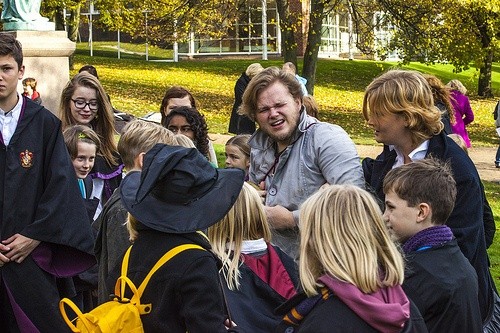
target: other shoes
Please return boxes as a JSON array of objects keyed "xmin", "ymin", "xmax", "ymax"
[{"xmin": 494, "ymin": 161, "xmax": 500, "ymax": 168}]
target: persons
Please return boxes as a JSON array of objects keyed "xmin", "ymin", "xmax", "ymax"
[
  {"xmin": 241, "ymin": 66, "xmax": 366, "ymax": 263},
  {"xmin": 164, "ymin": 106, "xmax": 210, "ymax": 161},
  {"xmin": 382, "ymin": 159, "xmax": 484, "ymax": 333},
  {"xmin": 160, "ymin": 87, "xmax": 218, "ymax": 168},
  {"xmin": 207, "ymin": 181, "xmax": 304, "ymax": 333},
  {"xmin": 362, "ymin": 70, "xmax": 495, "ymax": 325},
  {"xmin": 92, "ymin": 120, "xmax": 195, "ymax": 305},
  {"xmin": 60, "ymin": 65, "xmax": 126, "ymax": 225},
  {"xmin": 119, "ymin": 143, "xmax": 245, "ymax": 333},
  {"xmin": 494, "ymin": 100, "xmax": 500, "ymax": 167},
  {"xmin": 226, "ymin": 135, "xmax": 253, "ymax": 181},
  {"xmin": 228, "ymin": 63, "xmax": 264, "ymax": 134},
  {"xmin": 64, "ymin": 125, "xmax": 100, "ymax": 199},
  {"xmin": 274, "ymin": 184, "xmax": 429, "ymax": 333},
  {"xmin": 283, "ymin": 61, "xmax": 308, "ymax": 96},
  {"xmin": 22, "ymin": 78, "xmax": 42, "ymax": 106},
  {"xmin": 447, "ymin": 79, "xmax": 474, "ymax": 147},
  {"xmin": 0, "ymin": 33, "xmax": 99, "ymax": 333}
]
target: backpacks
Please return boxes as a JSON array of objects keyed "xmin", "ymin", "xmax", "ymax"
[
  {"xmin": 59, "ymin": 243, "xmax": 208, "ymax": 333},
  {"xmin": 492, "ymin": 101, "xmax": 499, "ymax": 120}
]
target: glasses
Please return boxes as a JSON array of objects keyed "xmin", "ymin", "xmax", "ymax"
[{"xmin": 71, "ymin": 97, "xmax": 100, "ymax": 110}]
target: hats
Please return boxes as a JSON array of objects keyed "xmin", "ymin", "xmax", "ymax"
[{"xmin": 119, "ymin": 143, "xmax": 246, "ymax": 234}]
